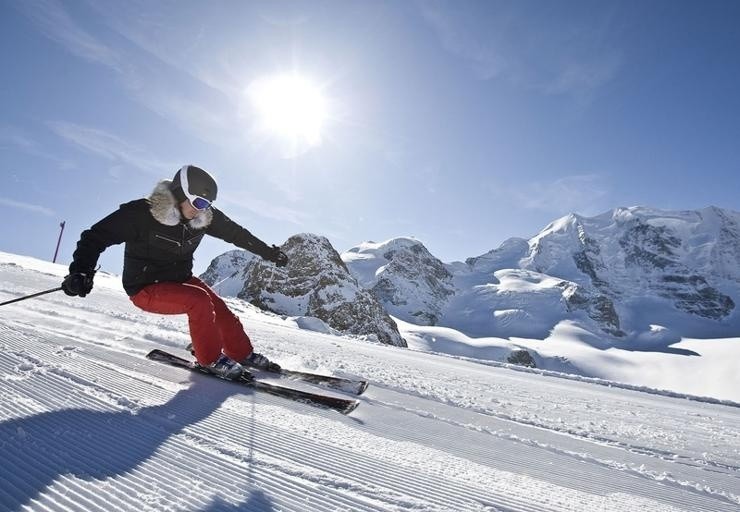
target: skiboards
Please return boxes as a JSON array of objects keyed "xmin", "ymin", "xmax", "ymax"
[{"xmin": 146, "ymin": 343, "xmax": 369, "ymax": 415}]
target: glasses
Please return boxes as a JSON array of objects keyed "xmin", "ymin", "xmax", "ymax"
[{"xmin": 188, "ymin": 194, "xmax": 213, "ymax": 211}]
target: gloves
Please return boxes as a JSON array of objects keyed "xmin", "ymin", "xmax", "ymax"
[
  {"xmin": 63, "ymin": 264, "xmax": 97, "ymax": 298},
  {"xmin": 270, "ymin": 244, "xmax": 289, "ymax": 268}
]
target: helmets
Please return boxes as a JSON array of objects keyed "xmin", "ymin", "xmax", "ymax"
[{"xmin": 170, "ymin": 166, "xmax": 217, "ymax": 204}]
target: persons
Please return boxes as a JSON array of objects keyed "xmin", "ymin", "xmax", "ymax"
[{"xmin": 61, "ymin": 162, "xmax": 290, "ymax": 382}]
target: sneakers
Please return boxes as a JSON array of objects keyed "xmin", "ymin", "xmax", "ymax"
[
  {"xmin": 239, "ymin": 352, "xmax": 270, "ymax": 371},
  {"xmin": 198, "ymin": 352, "xmax": 244, "ymax": 381}
]
